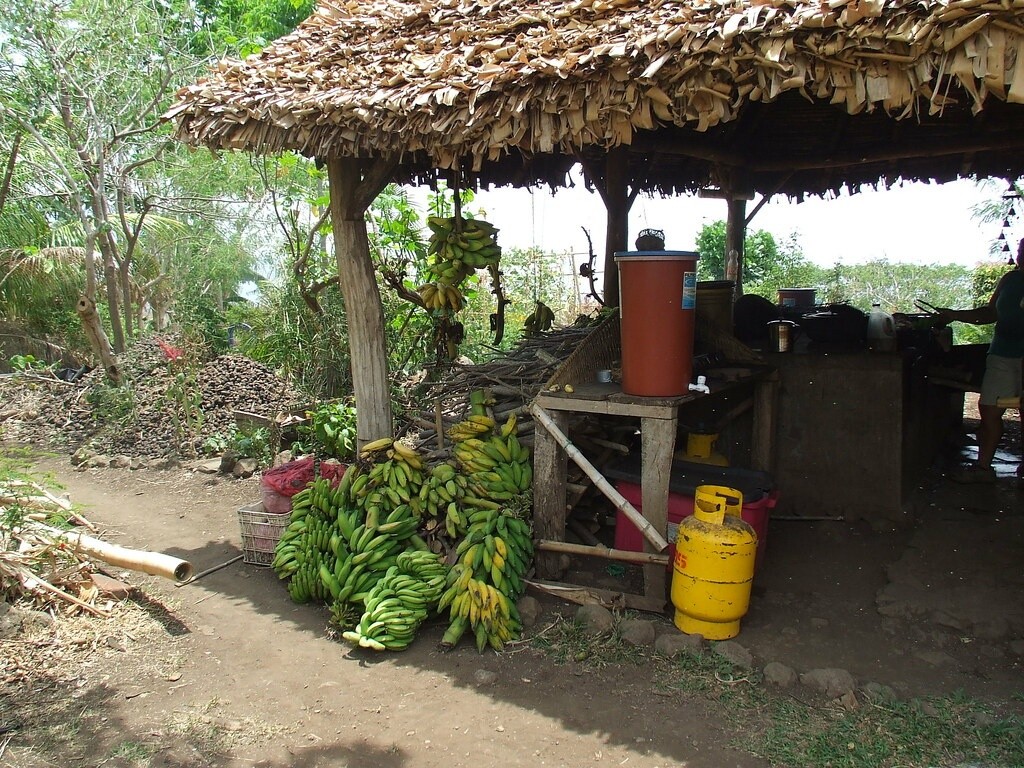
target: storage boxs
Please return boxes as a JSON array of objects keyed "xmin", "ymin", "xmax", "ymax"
[
  {"xmin": 601, "ymin": 450, "xmax": 783, "ymax": 572},
  {"xmin": 236, "ymin": 501, "xmax": 293, "ymax": 566}
]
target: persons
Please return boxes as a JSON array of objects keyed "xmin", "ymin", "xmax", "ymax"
[{"xmin": 935, "ymin": 237, "xmax": 1024, "ymax": 484}]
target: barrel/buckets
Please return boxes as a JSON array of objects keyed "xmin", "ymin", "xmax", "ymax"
[
  {"xmin": 613, "ymin": 251, "xmax": 700, "ymax": 396},
  {"xmin": 696, "ymin": 288, "xmax": 735, "ymax": 334}
]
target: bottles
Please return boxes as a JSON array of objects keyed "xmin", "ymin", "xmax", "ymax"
[{"xmin": 867, "ymin": 303, "xmax": 896, "ymax": 353}]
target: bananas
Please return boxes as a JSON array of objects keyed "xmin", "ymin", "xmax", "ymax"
[
  {"xmin": 271, "ymin": 414, "xmax": 537, "ymax": 653},
  {"xmin": 416, "ymin": 216, "xmax": 500, "ymax": 314},
  {"xmin": 549, "ymin": 383, "xmax": 574, "ymax": 393}
]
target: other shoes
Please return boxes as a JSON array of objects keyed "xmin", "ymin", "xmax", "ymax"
[{"xmin": 951, "ymin": 464, "xmax": 996, "ymax": 483}]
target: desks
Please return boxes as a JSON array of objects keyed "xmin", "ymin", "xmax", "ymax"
[{"xmin": 530, "ymin": 372, "xmax": 778, "ymax": 616}]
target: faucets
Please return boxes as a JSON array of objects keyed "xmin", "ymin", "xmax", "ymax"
[{"xmin": 689, "ymin": 373, "xmax": 710, "ymax": 395}]
